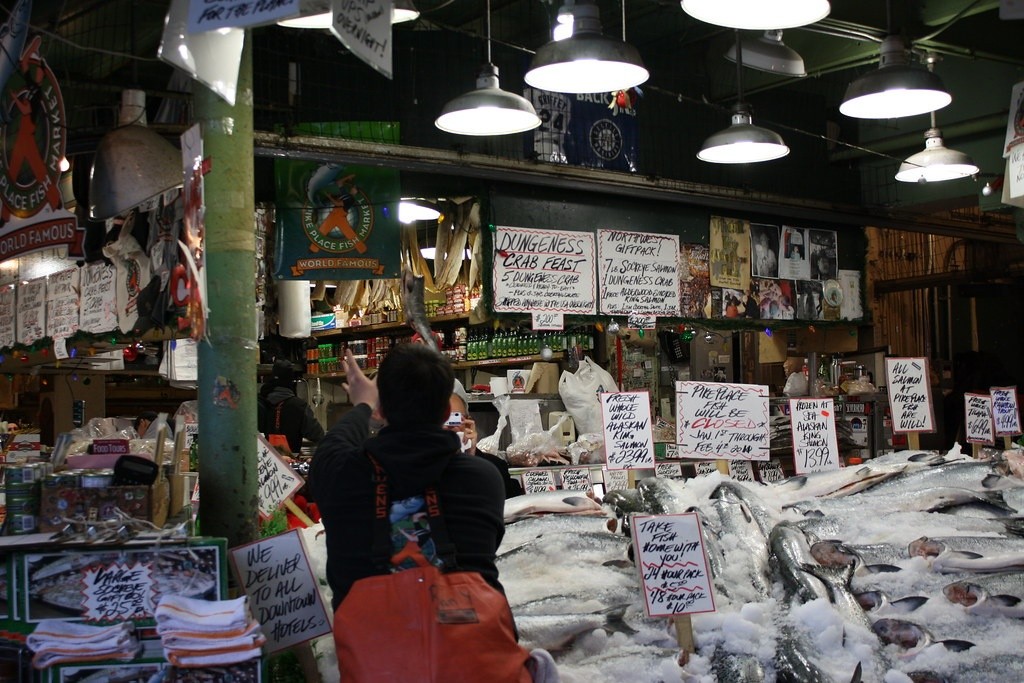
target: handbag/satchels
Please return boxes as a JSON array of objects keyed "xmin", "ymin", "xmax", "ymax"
[{"xmin": 558, "ymin": 355, "xmax": 619, "ymax": 437}]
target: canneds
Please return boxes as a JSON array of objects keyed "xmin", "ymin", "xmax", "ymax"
[
  {"xmin": 4, "ymin": 462, "xmax": 113, "ymax": 535},
  {"xmin": 305, "ymin": 346, "xmax": 320, "ymax": 376},
  {"xmin": 366, "ymin": 336, "xmax": 389, "ymax": 368},
  {"xmin": 435, "ymin": 326, "xmax": 467, "ymax": 362}
]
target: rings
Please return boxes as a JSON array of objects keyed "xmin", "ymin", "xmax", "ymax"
[{"xmin": 469, "ymin": 429, "xmax": 473, "ymax": 434}]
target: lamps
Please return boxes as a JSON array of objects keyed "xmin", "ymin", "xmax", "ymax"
[
  {"xmin": 275, "ymin": 0, "xmax": 421, "ymax": 29},
  {"xmin": 838, "ymin": 0, "xmax": 952, "ymax": 119},
  {"xmin": 721, "ymin": 27, "xmax": 807, "ymax": 77},
  {"xmin": 433, "ymin": 0, "xmax": 542, "ymax": 137},
  {"xmin": 522, "ymin": 0, "xmax": 650, "ymax": 94},
  {"xmin": 695, "ymin": 29, "xmax": 791, "ymax": 164},
  {"xmin": 680, "ymin": 0, "xmax": 831, "ymax": 30},
  {"xmin": 894, "ymin": 52, "xmax": 980, "ymax": 184}
]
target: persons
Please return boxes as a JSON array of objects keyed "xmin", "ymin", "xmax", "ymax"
[
  {"xmin": 308, "ymin": 343, "xmax": 521, "ymax": 639},
  {"xmin": 7, "ymin": 422, "xmax": 20, "ymax": 432},
  {"xmin": 443, "ymin": 379, "xmax": 525, "ymax": 497},
  {"xmin": 322, "ymin": 172, "xmax": 360, "ymax": 213},
  {"xmin": 679, "ymin": 233, "xmax": 831, "ymax": 317},
  {"xmin": 134, "ymin": 411, "xmax": 173, "ymax": 439},
  {"xmin": 258, "ymin": 358, "xmax": 325, "ymax": 452}
]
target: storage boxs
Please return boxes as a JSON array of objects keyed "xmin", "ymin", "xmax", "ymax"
[
  {"xmin": 88, "ymin": 349, "xmax": 124, "ymax": 370},
  {"xmin": 4, "ymin": 433, "xmax": 41, "ymax": 464},
  {"xmin": 506, "ymin": 369, "xmax": 531, "ymax": 393},
  {"xmin": 311, "ymin": 283, "xmax": 481, "ymax": 332}
]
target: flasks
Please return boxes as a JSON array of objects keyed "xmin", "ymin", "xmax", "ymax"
[{"xmin": 852, "ymin": 361, "xmax": 868, "ymax": 381}]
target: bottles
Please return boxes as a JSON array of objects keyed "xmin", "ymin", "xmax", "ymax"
[
  {"xmin": 466, "ymin": 324, "xmax": 594, "ymax": 361},
  {"xmin": 801, "ymin": 358, "xmax": 809, "ymax": 381},
  {"xmin": 818, "ymin": 352, "xmax": 843, "ymax": 389}
]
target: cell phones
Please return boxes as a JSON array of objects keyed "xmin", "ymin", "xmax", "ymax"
[{"xmin": 444, "ymin": 412, "xmax": 462, "ymax": 426}]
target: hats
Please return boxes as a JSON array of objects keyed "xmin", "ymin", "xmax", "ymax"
[{"xmin": 453, "ymin": 377, "xmax": 469, "ymax": 414}]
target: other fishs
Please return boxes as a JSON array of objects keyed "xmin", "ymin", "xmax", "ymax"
[{"xmin": 492, "ymin": 449, "xmax": 1024, "ymax": 683}]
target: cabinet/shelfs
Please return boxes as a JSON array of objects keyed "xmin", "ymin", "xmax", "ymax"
[{"xmin": 302, "ymin": 312, "xmax": 595, "ymax": 379}]
